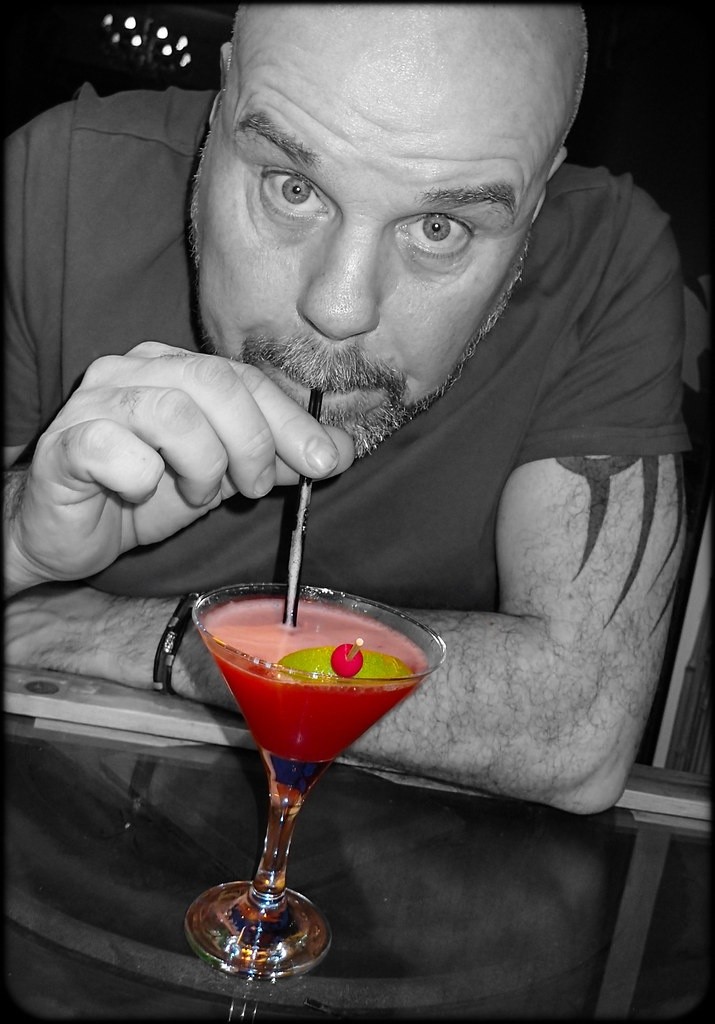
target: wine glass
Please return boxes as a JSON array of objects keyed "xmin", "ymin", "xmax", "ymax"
[{"xmin": 182, "ymin": 584, "xmax": 446, "ymax": 975}]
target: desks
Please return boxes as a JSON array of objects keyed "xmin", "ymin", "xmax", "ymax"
[{"xmin": 0, "ymin": 716, "xmax": 715, "ymax": 1024}]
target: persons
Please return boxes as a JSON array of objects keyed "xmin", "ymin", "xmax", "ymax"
[{"xmin": 0, "ymin": 0, "xmax": 694, "ymax": 815}]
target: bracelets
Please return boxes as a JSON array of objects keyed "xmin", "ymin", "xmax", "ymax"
[{"xmin": 148, "ymin": 586, "xmax": 210, "ymax": 704}]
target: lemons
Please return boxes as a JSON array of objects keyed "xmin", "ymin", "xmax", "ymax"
[{"xmin": 273, "ymin": 645, "xmax": 416, "ymax": 685}]
